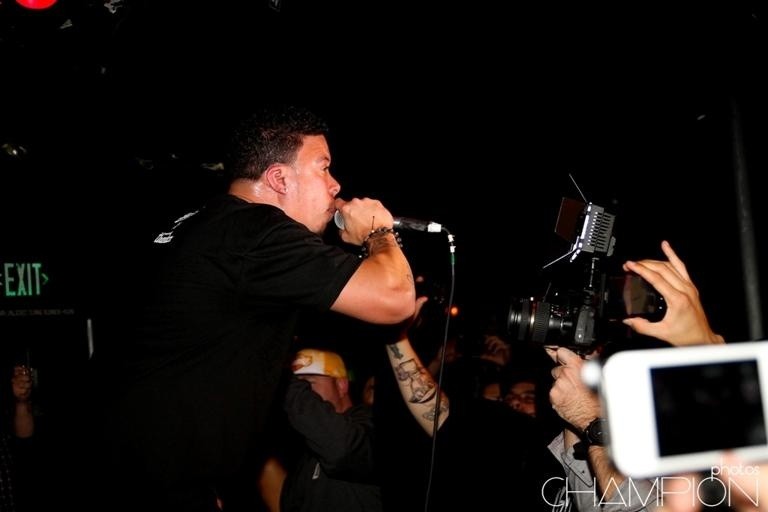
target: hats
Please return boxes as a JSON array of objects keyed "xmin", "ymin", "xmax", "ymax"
[{"xmin": 285, "ymin": 346, "xmax": 349, "ymax": 380}]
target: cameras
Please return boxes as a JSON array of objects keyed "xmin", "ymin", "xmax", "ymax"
[
  {"xmin": 506, "ymin": 199, "xmax": 668, "ymax": 356},
  {"xmin": 596, "ymin": 341, "xmax": 768, "ymax": 480}
]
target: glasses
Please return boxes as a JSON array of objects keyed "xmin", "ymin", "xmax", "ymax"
[{"xmin": 496, "ymin": 390, "xmax": 539, "ymax": 407}]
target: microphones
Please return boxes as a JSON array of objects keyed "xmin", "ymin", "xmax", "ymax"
[{"xmin": 333, "ymin": 208, "xmax": 443, "ymax": 239}]
